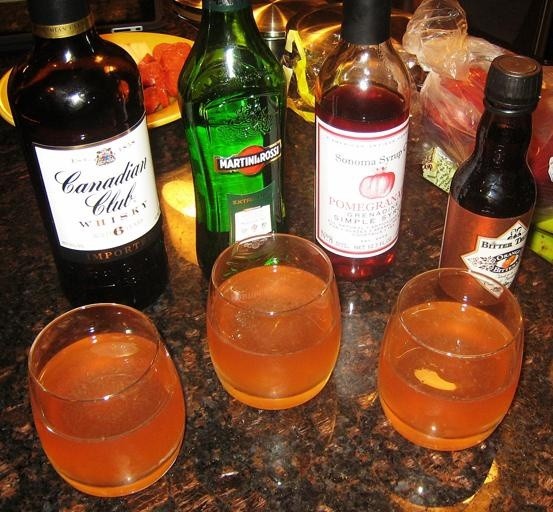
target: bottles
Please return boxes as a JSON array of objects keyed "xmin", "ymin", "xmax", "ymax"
[
  {"xmin": 434, "ymin": 51, "xmax": 551, "ymax": 313},
  {"xmin": 177, "ymin": 1, "xmax": 288, "ymax": 282},
  {"xmin": 8, "ymin": 2, "xmax": 176, "ymax": 312}
]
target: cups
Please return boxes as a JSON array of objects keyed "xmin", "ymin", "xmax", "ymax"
[
  {"xmin": 314, "ymin": 2, "xmax": 408, "ymax": 283},
  {"xmin": 25, "ymin": 300, "xmax": 190, "ymax": 502},
  {"xmin": 201, "ymin": 232, "xmax": 346, "ymax": 415},
  {"xmin": 377, "ymin": 268, "xmax": 525, "ymax": 456}
]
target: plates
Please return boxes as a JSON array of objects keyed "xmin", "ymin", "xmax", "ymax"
[{"xmin": 1, "ymin": 29, "xmax": 195, "ymax": 132}]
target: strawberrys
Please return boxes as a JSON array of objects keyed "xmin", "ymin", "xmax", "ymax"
[{"xmin": 138, "ymin": 43, "xmax": 193, "ymax": 115}]
